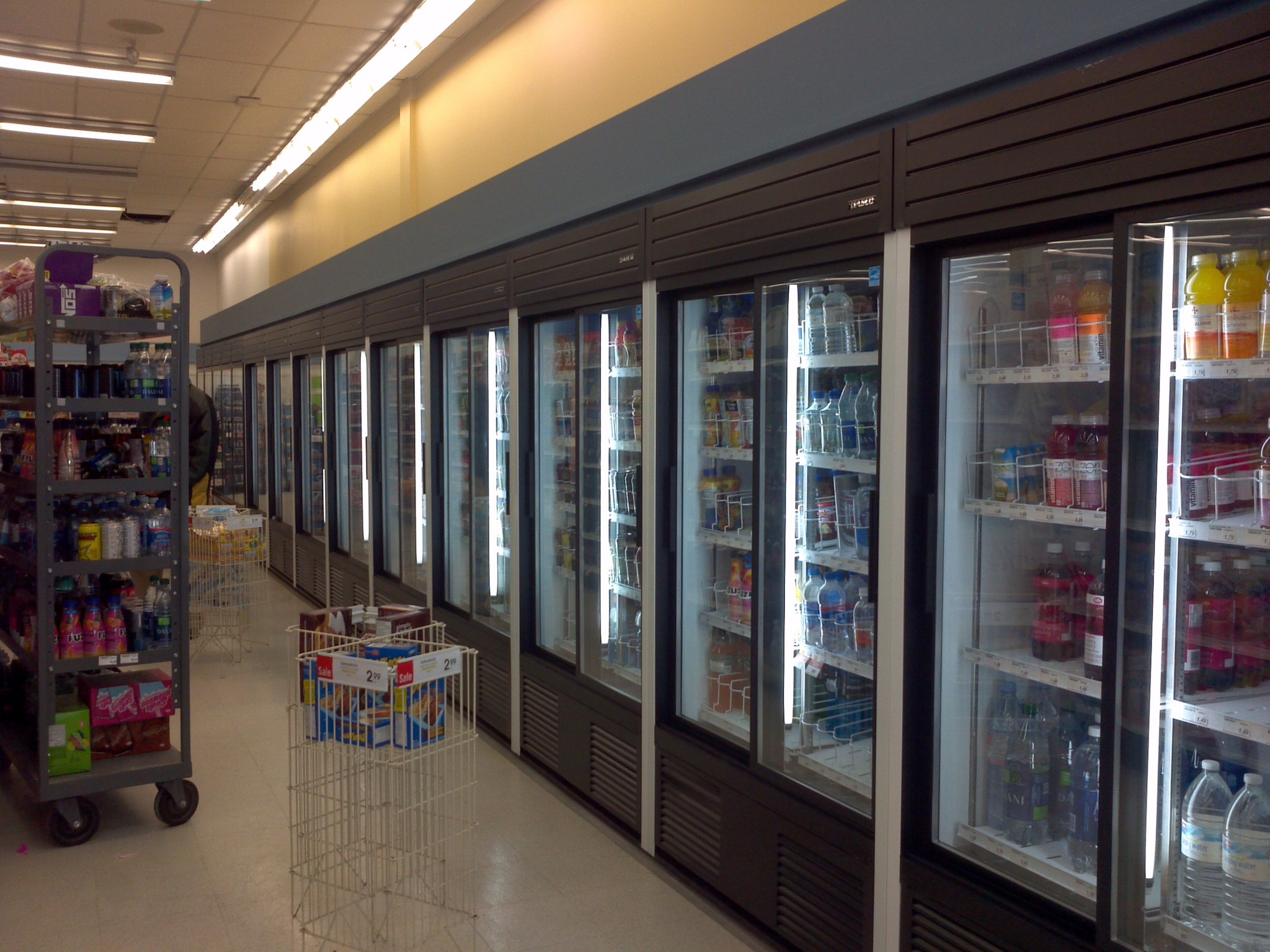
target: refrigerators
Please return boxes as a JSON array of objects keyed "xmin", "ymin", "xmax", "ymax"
[
  {"xmin": 654, "ymin": 234, "xmax": 885, "ymax": 951},
  {"xmin": 211, "ymin": 314, "xmax": 645, "ymax": 838},
  {"xmin": 899, "ymin": 194, "xmax": 1270, "ymax": 949}
]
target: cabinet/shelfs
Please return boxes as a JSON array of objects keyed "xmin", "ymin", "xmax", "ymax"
[{"xmin": 0, "ymin": 244, "xmax": 192, "ymax": 802}]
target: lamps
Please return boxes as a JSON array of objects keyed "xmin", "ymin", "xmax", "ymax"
[{"xmin": 0, "ymin": 43, "xmax": 176, "ymax": 236}]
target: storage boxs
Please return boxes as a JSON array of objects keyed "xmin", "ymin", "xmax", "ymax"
[
  {"xmin": 16, "ymin": 241, "xmax": 101, "ymax": 317},
  {"xmin": 304, "ymin": 643, "xmax": 443, "ymax": 749},
  {"xmin": 50, "ymin": 669, "xmax": 175, "ymax": 776}
]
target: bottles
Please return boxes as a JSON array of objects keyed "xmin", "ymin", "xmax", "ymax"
[
  {"xmin": 0, "ymin": 273, "xmax": 174, "ymax": 664},
  {"xmin": 981, "ymin": 248, "xmax": 1270, "ymax": 952},
  {"xmin": 188, "ymin": 505, "xmax": 194, "ymax": 529},
  {"xmin": 698, "ymin": 283, "xmax": 882, "ymax": 714}
]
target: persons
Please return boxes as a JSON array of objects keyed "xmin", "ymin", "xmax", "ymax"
[{"xmin": 140, "ymin": 377, "xmax": 219, "ymax": 512}]
